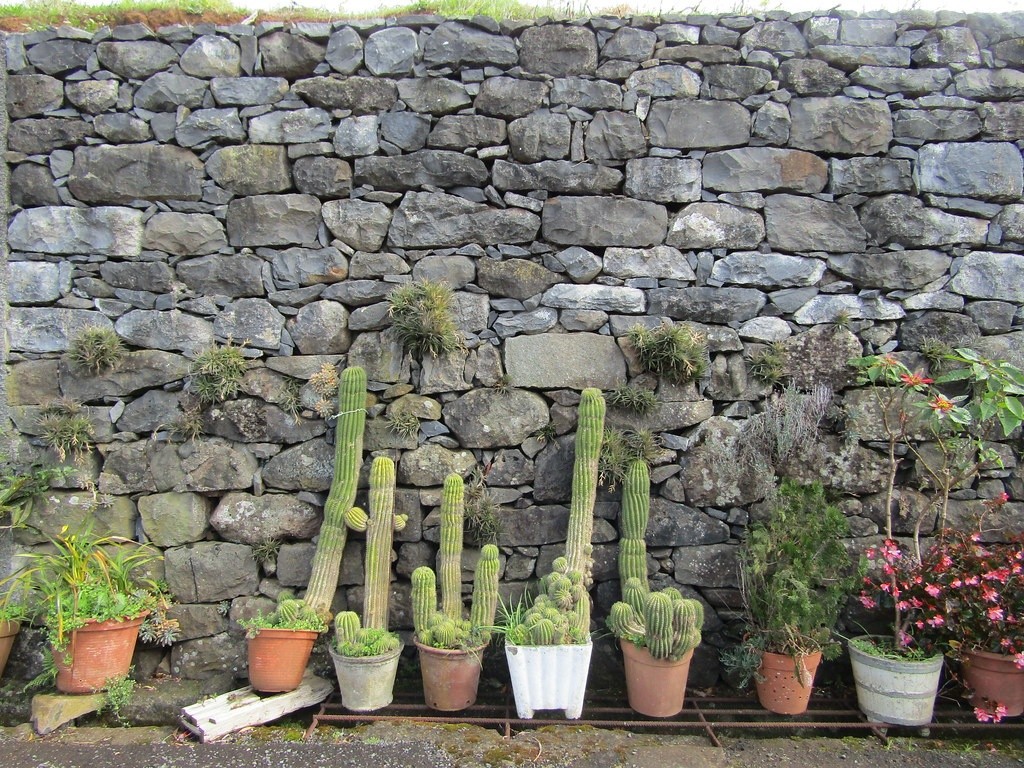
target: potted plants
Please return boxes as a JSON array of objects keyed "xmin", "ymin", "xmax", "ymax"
[
  {"xmin": 329, "ymin": 457, "xmax": 406, "ymax": 713},
  {"xmin": 720, "ymin": 477, "xmax": 867, "ymax": 715},
  {"xmin": 606, "ymin": 460, "xmax": 704, "ymax": 718},
  {"xmin": 0, "ymin": 516, "xmax": 182, "ymax": 696},
  {"xmin": 234, "ymin": 366, "xmax": 366, "ymax": 691},
  {"xmin": 477, "ymin": 388, "xmax": 605, "ymax": 718},
  {"xmin": 411, "ymin": 474, "xmax": 500, "ymax": 712},
  {"xmin": 0, "ymin": 603, "xmax": 34, "ymax": 678},
  {"xmin": 832, "ymin": 348, "xmax": 1024, "ymax": 727}
]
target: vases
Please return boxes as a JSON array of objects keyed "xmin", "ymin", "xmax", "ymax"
[{"xmin": 960, "ymin": 644, "xmax": 1024, "ymax": 717}]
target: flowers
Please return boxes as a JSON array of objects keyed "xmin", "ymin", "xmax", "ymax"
[{"xmin": 860, "ymin": 490, "xmax": 1024, "ymax": 723}]
